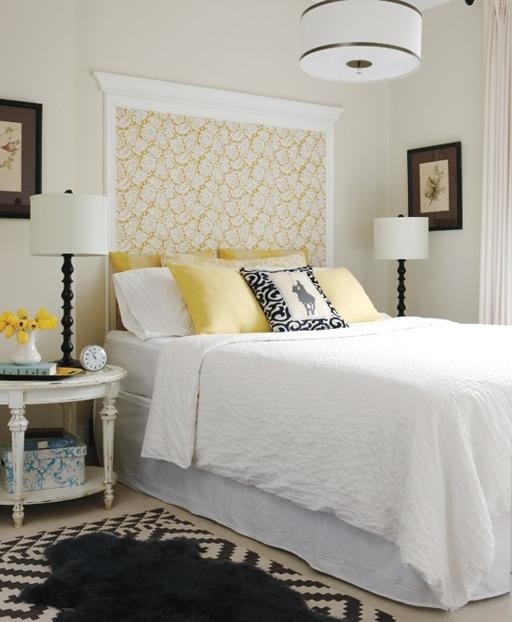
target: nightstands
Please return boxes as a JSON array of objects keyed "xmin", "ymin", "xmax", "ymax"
[{"xmin": 0, "ymin": 364, "xmax": 129, "ymax": 528}]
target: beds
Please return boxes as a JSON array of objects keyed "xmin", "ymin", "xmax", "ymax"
[{"xmin": 97, "ymin": 247, "xmax": 511, "ymax": 610}]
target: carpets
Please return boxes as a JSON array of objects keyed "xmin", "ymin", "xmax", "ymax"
[{"xmin": 0, "ymin": 509, "xmax": 398, "ymax": 622}]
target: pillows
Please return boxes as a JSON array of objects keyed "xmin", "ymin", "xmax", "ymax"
[
  {"xmin": 242, "ymin": 266, "xmax": 347, "ymax": 331},
  {"xmin": 161, "ymin": 254, "xmax": 307, "ymax": 267},
  {"xmin": 167, "ymin": 265, "xmax": 383, "ymax": 333}
]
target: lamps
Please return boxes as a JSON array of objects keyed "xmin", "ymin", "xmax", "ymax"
[
  {"xmin": 372, "ymin": 214, "xmax": 428, "ymax": 317},
  {"xmin": 30, "ymin": 190, "xmax": 108, "ymax": 373},
  {"xmin": 298, "ymin": 1, "xmax": 421, "ymax": 84}
]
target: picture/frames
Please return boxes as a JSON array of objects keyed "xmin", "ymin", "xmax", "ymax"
[
  {"xmin": 2, "ymin": 99, "xmax": 41, "ymax": 219},
  {"xmin": 406, "ymin": 141, "xmax": 462, "ymax": 230}
]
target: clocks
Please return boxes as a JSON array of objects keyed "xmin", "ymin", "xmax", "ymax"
[{"xmin": 79, "ymin": 345, "xmax": 107, "ymax": 371}]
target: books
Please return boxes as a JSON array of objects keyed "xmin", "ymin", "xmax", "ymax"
[
  {"xmin": 0, "ymin": 363, "xmax": 57, "ymax": 375},
  {"xmin": 26, "ymin": 427, "xmax": 65, "ymax": 438},
  {"xmin": 24, "ymin": 430, "xmax": 80, "ymax": 451}
]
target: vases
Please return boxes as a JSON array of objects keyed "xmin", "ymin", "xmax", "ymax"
[{"xmin": 14, "ymin": 331, "xmax": 41, "ymax": 364}]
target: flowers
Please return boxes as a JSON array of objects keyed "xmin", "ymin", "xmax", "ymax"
[{"xmin": 0, "ymin": 308, "xmax": 57, "ymax": 341}]
